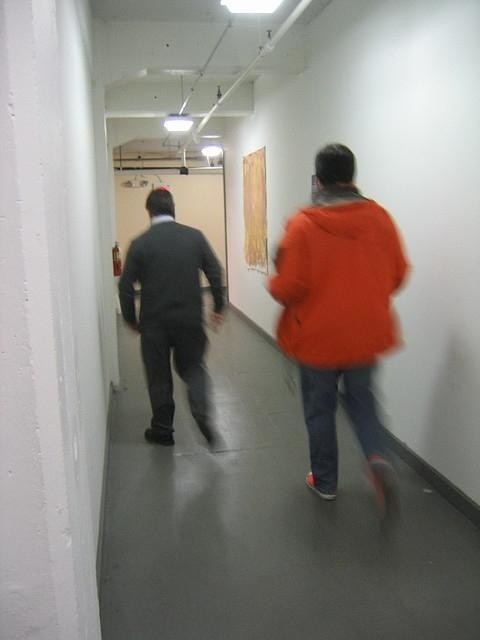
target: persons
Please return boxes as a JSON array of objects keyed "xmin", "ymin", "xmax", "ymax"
[
  {"xmin": 264, "ymin": 144, "xmax": 408, "ymax": 513},
  {"xmin": 118, "ymin": 187, "xmax": 226, "ymax": 449}
]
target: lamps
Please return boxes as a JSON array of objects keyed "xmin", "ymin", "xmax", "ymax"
[
  {"xmin": 200, "ymin": 143, "xmax": 222, "ymax": 155},
  {"xmin": 161, "ymin": 112, "xmax": 194, "ymax": 132}
]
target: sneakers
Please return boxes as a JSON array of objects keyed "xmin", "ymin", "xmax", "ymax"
[
  {"xmin": 370, "ymin": 454, "xmax": 398, "ymax": 495},
  {"xmin": 145, "ymin": 428, "xmax": 174, "ymax": 445},
  {"xmin": 306, "ymin": 472, "xmax": 337, "ymax": 500}
]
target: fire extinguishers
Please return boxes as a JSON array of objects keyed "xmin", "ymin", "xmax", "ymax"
[{"xmin": 112, "ymin": 241, "xmax": 122, "ymax": 276}]
